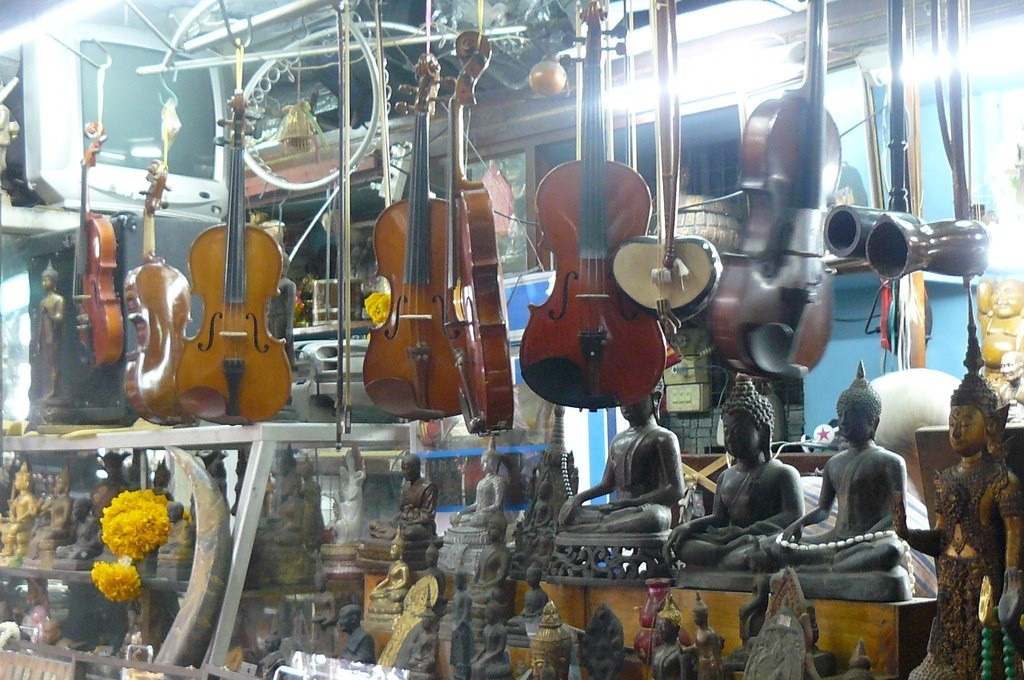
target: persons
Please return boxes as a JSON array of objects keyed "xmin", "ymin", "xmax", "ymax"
[
  {"xmin": 468, "ymin": 601, "xmax": 514, "ymax": 680},
  {"xmin": 457, "ymin": 513, "xmax": 512, "ymax": 643},
  {"xmin": 433, "ymin": 434, "xmax": 505, "ymax": 580},
  {"xmin": 395, "ymin": 586, "xmax": 440, "ymax": 680},
  {"xmin": 544, "ymin": 375, "xmax": 682, "ymax": 575},
  {"xmin": 907, "ymin": 291, "xmax": 1021, "ymax": 679},
  {"xmin": 353, "ymin": 450, "xmax": 438, "ymax": 573},
  {"xmin": 2, "ymin": 442, "xmax": 337, "ymax": 680},
  {"xmin": 33, "ymin": 256, "xmax": 68, "ymax": 404},
  {"xmin": 841, "ymin": 638, "xmax": 875, "ymax": 679},
  {"xmin": 437, "ymin": 572, "xmax": 470, "ymax": 642},
  {"xmin": 339, "ymin": 604, "xmax": 375, "ymax": 665},
  {"xmin": 650, "ymin": 589, "xmax": 683, "ymax": 680},
  {"xmin": 662, "ymin": 372, "xmax": 800, "ymax": 589},
  {"xmin": 775, "ymin": 359, "xmax": 911, "ymax": 607},
  {"xmin": 693, "ymin": 590, "xmax": 723, "ymax": 680},
  {"xmin": 995, "ymin": 352, "xmax": 1023, "ymax": 426},
  {"xmin": 364, "ymin": 525, "xmax": 411, "ymax": 630},
  {"xmin": 973, "ymin": 278, "xmax": 1024, "ymax": 392},
  {"xmin": 502, "ymin": 562, "xmax": 548, "ymax": 648},
  {"xmin": 527, "ymin": 599, "xmax": 570, "ymax": 680}
]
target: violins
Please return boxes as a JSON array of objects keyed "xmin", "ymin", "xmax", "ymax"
[{"xmin": 71, "ymin": 1, "xmax": 844, "ymax": 438}]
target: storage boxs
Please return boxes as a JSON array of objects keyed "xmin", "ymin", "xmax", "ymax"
[{"xmin": 48, "ymin": 0, "xmax": 988, "ymax": 435}]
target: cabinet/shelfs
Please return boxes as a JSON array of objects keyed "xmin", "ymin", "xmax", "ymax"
[{"xmin": 1, "ymin": 423, "xmax": 937, "ymax": 680}]
